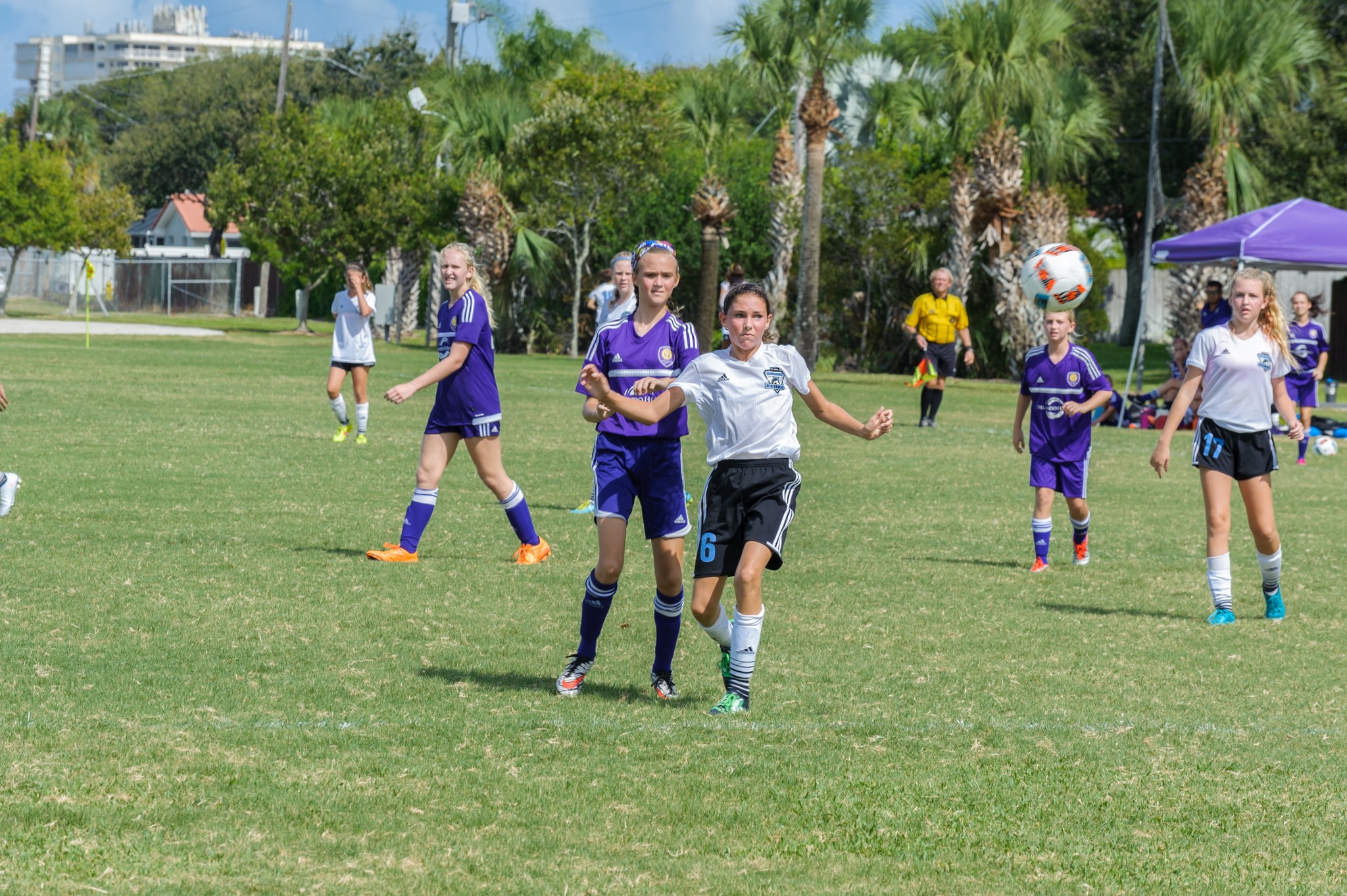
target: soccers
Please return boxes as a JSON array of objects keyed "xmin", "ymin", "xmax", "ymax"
[
  {"xmin": 1017, "ymin": 241, "xmax": 1093, "ymax": 311},
  {"xmin": 1314, "ymin": 435, "xmax": 1338, "ymax": 458}
]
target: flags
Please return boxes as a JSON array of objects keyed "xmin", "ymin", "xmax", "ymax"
[{"xmin": 904, "ymin": 357, "xmax": 938, "ymax": 387}]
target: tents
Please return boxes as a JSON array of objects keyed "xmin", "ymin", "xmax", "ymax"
[{"xmin": 1118, "ymin": 197, "xmax": 1347, "ymax": 427}]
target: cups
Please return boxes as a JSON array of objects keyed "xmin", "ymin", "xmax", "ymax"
[{"xmin": 1326, "ymin": 378, "xmax": 1337, "ymax": 403}]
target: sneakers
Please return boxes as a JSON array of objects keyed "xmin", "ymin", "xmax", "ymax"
[
  {"xmin": 367, "ymin": 542, "xmax": 419, "ymax": 564},
  {"xmin": 1072, "ymin": 534, "xmax": 1090, "ymax": 567},
  {"xmin": 650, "ymin": 670, "xmax": 678, "ymax": 700},
  {"xmin": 512, "ymin": 536, "xmax": 551, "ymax": 565},
  {"xmin": 0, "ymin": 472, "xmax": 21, "ymax": 518},
  {"xmin": 708, "ymin": 687, "xmax": 749, "ymax": 717},
  {"xmin": 1029, "ymin": 556, "xmax": 1050, "ymax": 573},
  {"xmin": 355, "ymin": 433, "xmax": 366, "ymax": 445},
  {"xmin": 332, "ymin": 420, "xmax": 354, "ymax": 443},
  {"xmin": 1206, "ymin": 608, "xmax": 1236, "ymax": 627},
  {"xmin": 1261, "ymin": 582, "xmax": 1285, "ymax": 620},
  {"xmin": 684, "ymin": 491, "xmax": 693, "ymax": 507},
  {"xmin": 569, "ymin": 499, "xmax": 595, "ymax": 514},
  {"xmin": 555, "ymin": 653, "xmax": 595, "ymax": 698},
  {"xmin": 719, "ymin": 619, "xmax": 737, "ymax": 691}
]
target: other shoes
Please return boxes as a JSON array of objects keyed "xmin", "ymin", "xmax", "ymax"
[{"xmin": 1297, "ymin": 458, "xmax": 1306, "ymax": 465}]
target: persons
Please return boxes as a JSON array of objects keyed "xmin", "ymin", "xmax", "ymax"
[
  {"xmin": 557, "ymin": 240, "xmax": 699, "ymax": 701},
  {"xmin": 327, "ymin": 263, "xmax": 376, "ymax": 444},
  {"xmin": 366, "ymin": 243, "xmax": 552, "ymax": 566},
  {"xmin": 1093, "ymin": 280, "xmax": 1234, "ymax": 426},
  {"xmin": 719, "ymin": 263, "xmax": 746, "ymax": 349},
  {"xmin": 570, "ymin": 252, "xmax": 694, "ymax": 513},
  {"xmin": 905, "ymin": 267, "xmax": 974, "ymax": 429},
  {"xmin": 1013, "ymin": 308, "xmax": 1112, "ymax": 572},
  {"xmin": 1149, "ymin": 268, "xmax": 1305, "ymax": 626},
  {"xmin": 0, "ymin": 382, "xmax": 21, "ymax": 515},
  {"xmin": 580, "ymin": 283, "xmax": 893, "ymax": 713},
  {"xmin": 1284, "ymin": 292, "xmax": 1329, "ymax": 463}
]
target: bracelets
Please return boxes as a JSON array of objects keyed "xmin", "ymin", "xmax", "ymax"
[
  {"xmin": 912, "ymin": 332, "xmax": 918, "ymax": 341},
  {"xmin": 964, "ymin": 346, "xmax": 974, "ymax": 351}
]
